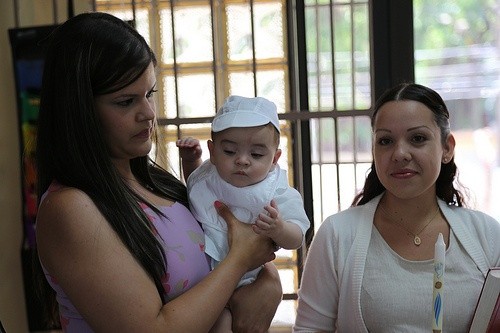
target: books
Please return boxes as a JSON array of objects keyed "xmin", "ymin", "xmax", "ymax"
[{"xmin": 468, "ymin": 267, "xmax": 499, "ymax": 333}]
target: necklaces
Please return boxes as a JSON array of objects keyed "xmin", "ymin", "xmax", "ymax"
[{"xmin": 378, "ymin": 202, "xmax": 439, "ymax": 246}]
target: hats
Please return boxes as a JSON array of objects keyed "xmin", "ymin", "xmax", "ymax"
[{"xmin": 210, "ymin": 95, "xmax": 280, "ymax": 134}]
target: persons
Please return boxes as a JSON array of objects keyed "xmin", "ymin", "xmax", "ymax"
[
  {"xmin": 177, "ymin": 95, "xmax": 312, "ymax": 333},
  {"xmin": 292, "ymin": 85, "xmax": 500, "ymax": 333},
  {"xmin": 31, "ymin": 11, "xmax": 283, "ymax": 333}
]
